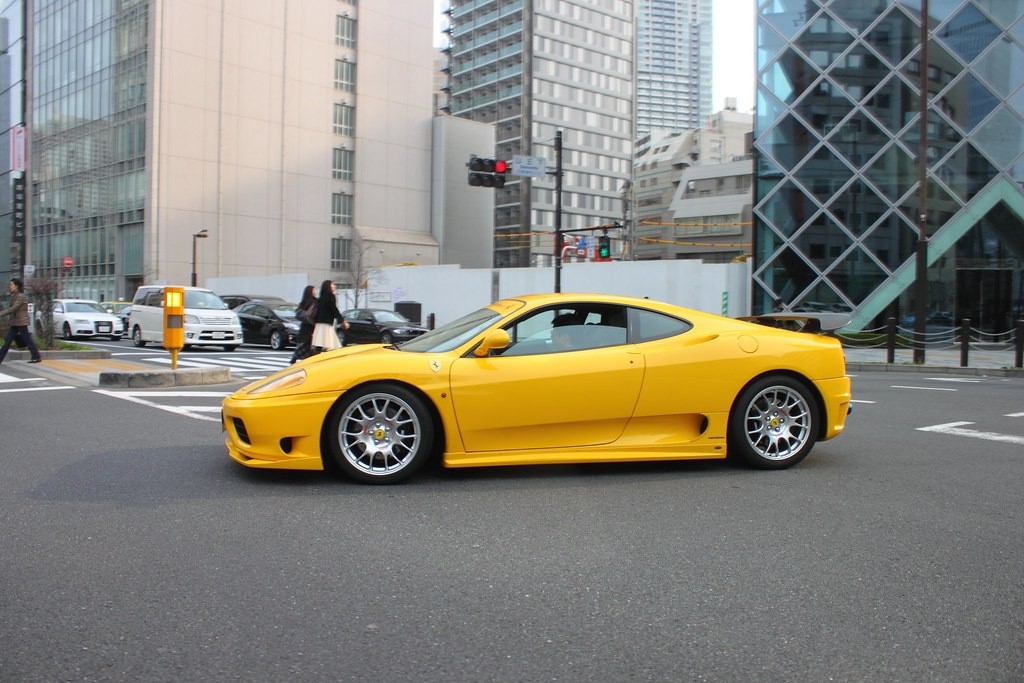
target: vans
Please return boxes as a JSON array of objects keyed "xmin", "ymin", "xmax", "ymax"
[{"xmin": 128, "ymin": 283, "xmax": 244, "ymax": 351}]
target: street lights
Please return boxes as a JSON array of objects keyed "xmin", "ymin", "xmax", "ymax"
[{"xmin": 191, "ymin": 228, "xmax": 209, "ymax": 287}]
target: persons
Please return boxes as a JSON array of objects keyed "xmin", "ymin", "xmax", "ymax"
[
  {"xmin": 311, "ymin": 280, "xmax": 350, "ymax": 352},
  {"xmin": 290, "ymin": 286, "xmax": 319, "ymax": 364},
  {"xmin": 0, "ymin": 278, "xmax": 41, "ymax": 365},
  {"xmin": 551, "ymin": 314, "xmax": 578, "ymax": 353}
]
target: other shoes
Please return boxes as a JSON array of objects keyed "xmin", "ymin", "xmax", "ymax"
[
  {"xmin": 28, "ymin": 359, "xmax": 41, "ymax": 363},
  {"xmin": 290, "ymin": 357, "xmax": 297, "ymax": 364}
]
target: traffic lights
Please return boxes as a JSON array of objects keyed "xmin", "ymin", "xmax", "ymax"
[
  {"xmin": 469, "ymin": 155, "xmax": 506, "ymax": 174},
  {"xmin": 598, "ymin": 236, "xmax": 610, "ymax": 261},
  {"xmin": 466, "ymin": 172, "xmax": 505, "ymax": 189}
]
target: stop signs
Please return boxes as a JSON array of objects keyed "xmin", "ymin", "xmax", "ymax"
[{"xmin": 63, "ymin": 255, "xmax": 74, "ymax": 270}]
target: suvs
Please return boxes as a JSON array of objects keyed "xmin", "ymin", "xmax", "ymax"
[{"xmin": 219, "ymin": 293, "xmax": 282, "ymax": 309}]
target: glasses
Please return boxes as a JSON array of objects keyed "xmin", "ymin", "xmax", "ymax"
[{"xmin": 551, "ymin": 330, "xmax": 559, "ymax": 335}]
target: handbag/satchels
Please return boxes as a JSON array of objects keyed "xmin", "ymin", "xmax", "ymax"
[{"xmin": 295, "ymin": 301, "xmax": 318, "ymax": 326}]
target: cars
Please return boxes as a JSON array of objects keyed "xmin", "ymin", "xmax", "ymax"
[
  {"xmin": 229, "ymin": 299, "xmax": 303, "ymax": 349},
  {"xmin": 219, "ymin": 293, "xmax": 854, "ymax": 485},
  {"xmin": 114, "ymin": 305, "xmax": 134, "ymax": 336},
  {"xmin": 33, "ymin": 298, "xmax": 124, "ymax": 340},
  {"xmin": 335, "ymin": 308, "xmax": 429, "ymax": 343},
  {"xmin": 100, "ymin": 299, "xmax": 132, "ymax": 316}
]
